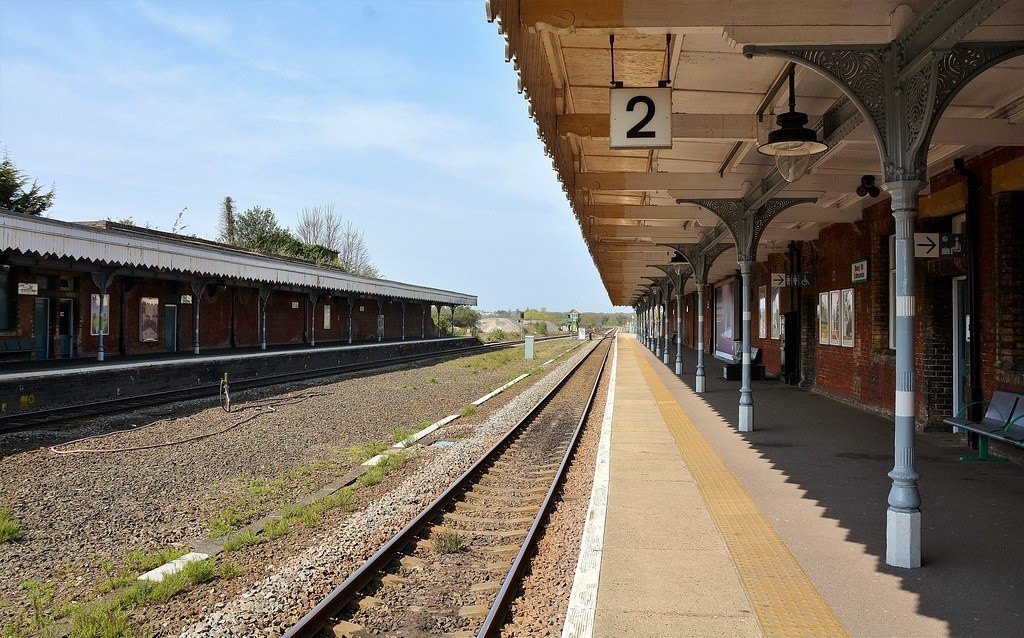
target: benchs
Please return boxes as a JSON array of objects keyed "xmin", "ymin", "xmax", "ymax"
[
  {"xmin": 943, "ymin": 390, "xmax": 1024, "ymax": 462},
  {"xmin": 0, "ymin": 339, "xmax": 45, "ymax": 361},
  {"xmin": 731, "ymin": 344, "xmax": 762, "ymax": 366}
]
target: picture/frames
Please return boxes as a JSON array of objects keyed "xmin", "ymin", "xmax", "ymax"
[
  {"xmin": 818, "ymin": 291, "xmax": 829, "ymax": 346},
  {"xmin": 841, "ymin": 288, "xmax": 855, "ymax": 348},
  {"xmin": 829, "ymin": 290, "xmax": 841, "ymax": 347}
]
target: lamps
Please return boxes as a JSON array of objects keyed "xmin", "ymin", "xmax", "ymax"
[
  {"xmin": 757, "ymin": 66, "xmax": 829, "ymax": 183},
  {"xmin": 668, "ymin": 250, "xmax": 691, "ymax": 275},
  {"xmin": 649, "ymin": 281, "xmax": 661, "ymax": 294}
]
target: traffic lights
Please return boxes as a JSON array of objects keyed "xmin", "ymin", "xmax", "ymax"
[{"xmin": 520, "ymin": 312, "xmax": 524, "ymax": 319}]
